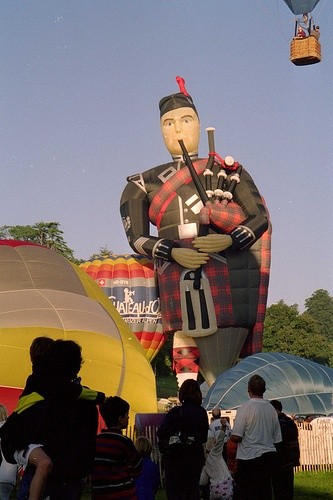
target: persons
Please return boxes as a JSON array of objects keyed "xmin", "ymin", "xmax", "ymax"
[
  {"xmin": 270, "ymin": 400, "xmax": 300, "ymax": 500},
  {"xmin": 118, "ymin": 75, "xmax": 269, "ymax": 387},
  {"xmin": 0, "ymin": 336, "xmax": 301, "ymax": 500},
  {"xmin": 133, "ymin": 436, "xmax": 160, "ymax": 500},
  {"xmin": 207, "ymin": 408, "xmax": 230, "ymax": 463},
  {"xmin": 0, "ymin": 336, "xmax": 108, "ymax": 500},
  {"xmin": 158, "ymin": 379, "xmax": 209, "ymax": 500},
  {"xmin": 230, "ymin": 374, "xmax": 282, "ymax": 500},
  {"xmin": 199, "ymin": 417, "xmax": 234, "ymax": 500},
  {"xmin": 16, "ymin": 339, "xmax": 83, "ymax": 500},
  {"xmin": 92, "ymin": 395, "xmax": 141, "ymax": 500},
  {"xmin": 0, "ymin": 403, "xmax": 16, "ymax": 500}
]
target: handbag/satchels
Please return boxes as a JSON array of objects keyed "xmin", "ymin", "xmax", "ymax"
[{"xmin": 167, "ymin": 406, "xmax": 195, "ymax": 448}]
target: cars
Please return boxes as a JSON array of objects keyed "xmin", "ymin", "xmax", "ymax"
[{"xmin": 293, "ymin": 413, "xmax": 326, "ymax": 425}]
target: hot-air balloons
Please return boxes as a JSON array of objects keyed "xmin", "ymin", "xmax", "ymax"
[
  {"xmin": 201, "ymin": 352, "xmax": 333, "ymax": 418},
  {"xmin": 0, "ymin": 239, "xmax": 158, "ymax": 447},
  {"xmin": 77, "ymin": 253, "xmax": 170, "ymax": 363},
  {"xmin": 119, "ymin": 75, "xmax": 273, "ymax": 388},
  {"xmin": 283, "ymin": 0, "xmax": 322, "ymax": 66}
]
